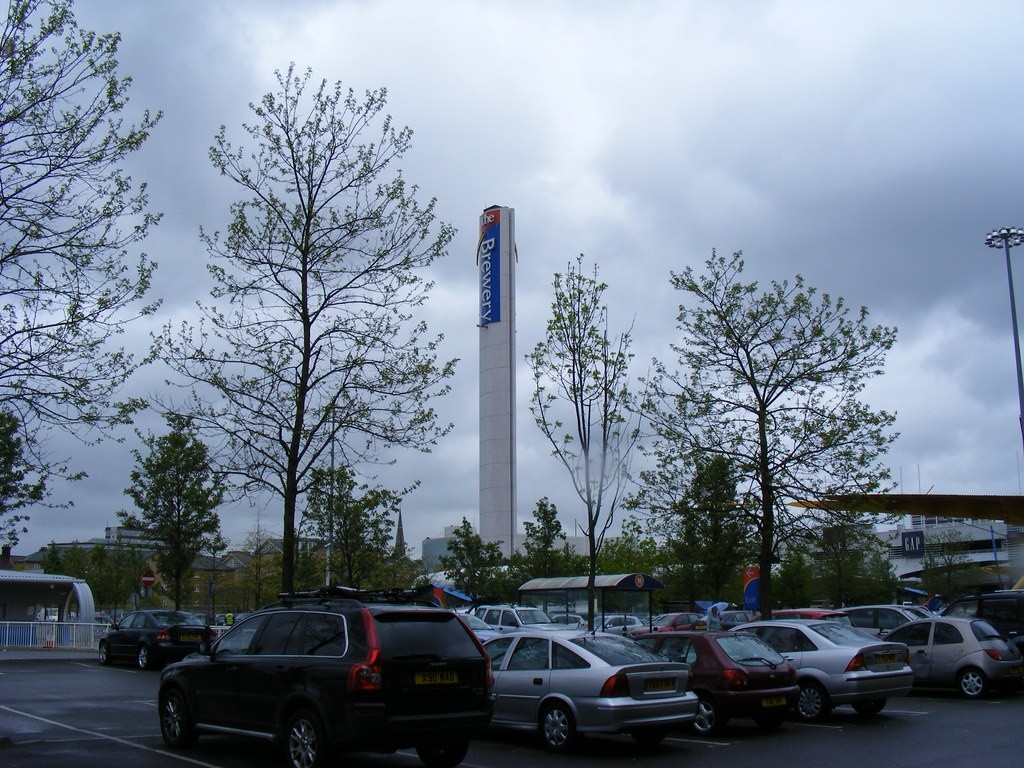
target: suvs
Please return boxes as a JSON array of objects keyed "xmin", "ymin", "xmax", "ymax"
[
  {"xmin": 941, "ymin": 589, "xmax": 1024, "ymax": 657},
  {"xmin": 157, "ymin": 588, "xmax": 497, "ymax": 768}
]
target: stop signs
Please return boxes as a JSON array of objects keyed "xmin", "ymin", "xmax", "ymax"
[{"xmin": 142, "ymin": 572, "xmax": 155, "ymax": 587}]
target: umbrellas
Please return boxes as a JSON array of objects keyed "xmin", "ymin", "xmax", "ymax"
[{"xmin": 707, "ymin": 602, "xmax": 729, "ymax": 616}]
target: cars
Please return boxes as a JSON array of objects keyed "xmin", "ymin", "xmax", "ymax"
[
  {"xmin": 36, "ymin": 608, "xmax": 251, "ymax": 639},
  {"xmin": 748, "ymin": 608, "xmax": 856, "ymax": 629},
  {"xmin": 879, "ymin": 615, "xmax": 1024, "ymax": 697},
  {"xmin": 629, "ymin": 631, "xmax": 800, "ymax": 734},
  {"xmin": 98, "ymin": 609, "xmax": 217, "ymax": 670},
  {"xmin": 479, "ymin": 629, "xmax": 699, "ymax": 751},
  {"xmin": 836, "ymin": 604, "xmax": 936, "ymax": 639},
  {"xmin": 453, "ymin": 602, "xmax": 759, "ymax": 644},
  {"xmin": 729, "ymin": 618, "xmax": 915, "ymax": 723}
]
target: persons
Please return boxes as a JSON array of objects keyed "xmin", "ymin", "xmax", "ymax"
[
  {"xmin": 225, "ymin": 610, "xmax": 234, "ymax": 626},
  {"xmin": 706, "ymin": 606, "xmax": 721, "ymax": 632}
]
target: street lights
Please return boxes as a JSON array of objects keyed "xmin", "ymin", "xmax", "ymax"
[{"xmin": 983, "ymin": 227, "xmax": 1024, "ymax": 439}]
